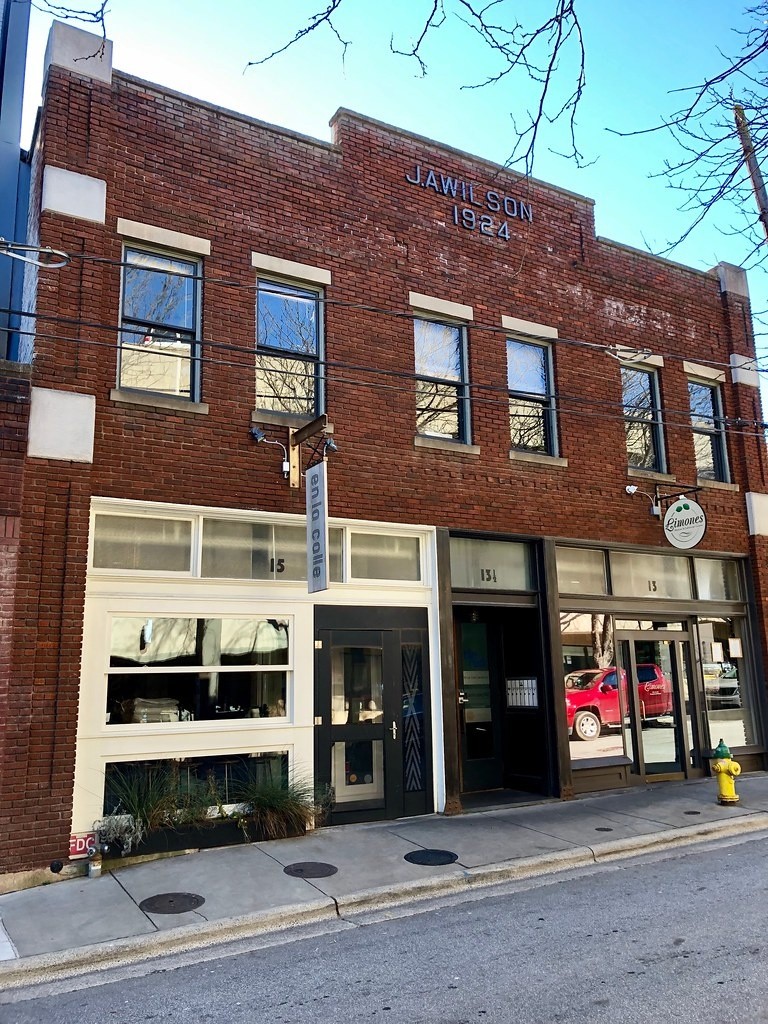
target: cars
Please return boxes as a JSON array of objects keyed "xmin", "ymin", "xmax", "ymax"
[
  {"xmin": 565, "ymin": 663, "xmax": 671, "ymax": 744},
  {"xmin": 706, "ymin": 667, "xmax": 739, "ymax": 709}
]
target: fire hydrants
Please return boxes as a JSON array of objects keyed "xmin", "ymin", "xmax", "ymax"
[{"xmin": 712, "ymin": 739, "xmax": 742, "ymax": 805}]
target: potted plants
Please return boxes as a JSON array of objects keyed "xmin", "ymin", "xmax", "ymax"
[{"xmin": 98, "ymin": 761, "xmax": 335, "ymax": 858}]
